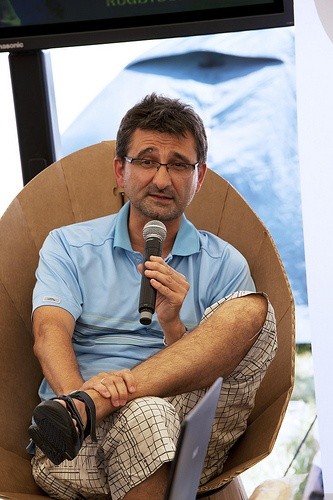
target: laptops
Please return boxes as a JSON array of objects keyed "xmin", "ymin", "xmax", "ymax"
[{"xmin": 164, "ymin": 377, "xmax": 223, "ymax": 500}]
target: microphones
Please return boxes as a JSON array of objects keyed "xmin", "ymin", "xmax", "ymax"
[{"xmin": 138, "ymin": 220, "xmax": 167, "ymax": 324}]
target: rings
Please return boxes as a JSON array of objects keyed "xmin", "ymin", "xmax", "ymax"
[{"xmin": 100, "ymin": 377, "xmax": 106, "ymax": 383}]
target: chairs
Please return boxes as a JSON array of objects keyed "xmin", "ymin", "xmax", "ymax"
[{"xmin": 0, "ymin": 141, "xmax": 295, "ymax": 500}]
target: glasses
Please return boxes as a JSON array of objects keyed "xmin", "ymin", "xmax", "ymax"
[{"xmin": 124, "ymin": 157, "xmax": 200, "ymax": 178}]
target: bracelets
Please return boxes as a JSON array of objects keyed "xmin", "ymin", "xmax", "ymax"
[{"xmin": 163, "ymin": 336, "xmax": 167, "ymax": 348}]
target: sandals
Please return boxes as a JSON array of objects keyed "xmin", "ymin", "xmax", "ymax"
[{"xmin": 27, "ymin": 390, "xmax": 98, "ymax": 466}]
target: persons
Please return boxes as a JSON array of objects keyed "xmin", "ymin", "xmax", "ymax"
[{"xmin": 28, "ymin": 92, "xmax": 275, "ymax": 500}]
